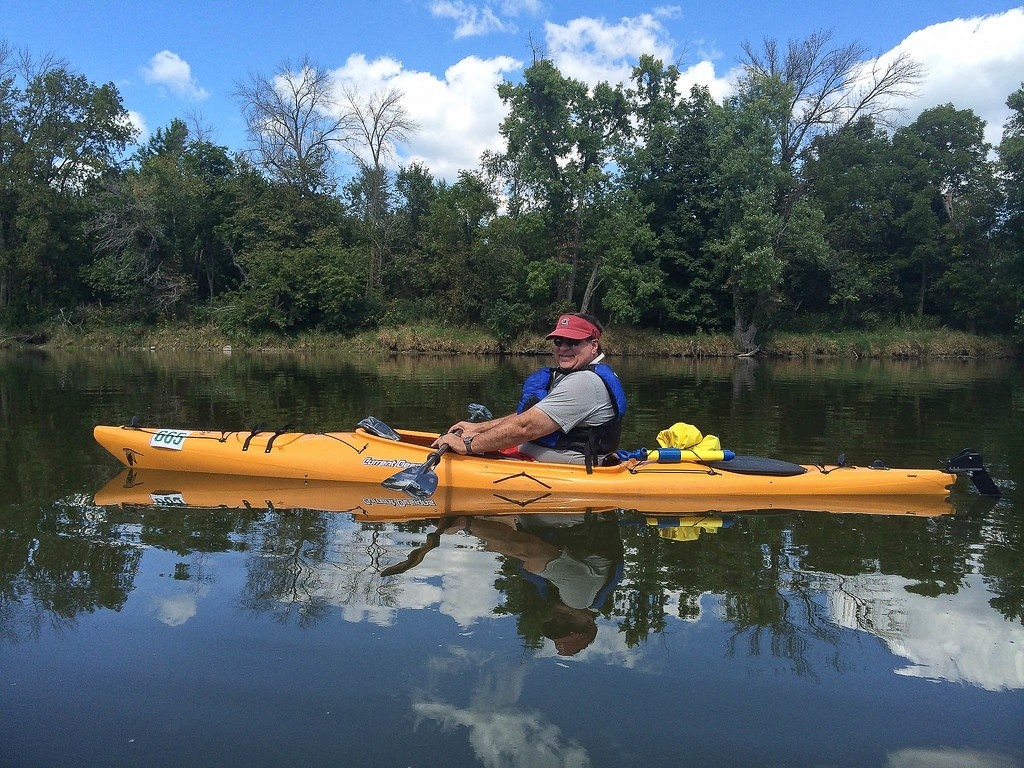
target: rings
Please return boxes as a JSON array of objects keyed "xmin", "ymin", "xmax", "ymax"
[{"xmin": 441, "ymin": 436, "xmax": 444, "ymax": 439}]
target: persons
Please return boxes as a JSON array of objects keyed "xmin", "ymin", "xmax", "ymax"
[
  {"xmin": 444, "ymin": 510, "xmax": 624, "ymax": 656},
  {"xmin": 431, "ymin": 313, "xmax": 626, "ymax": 467}
]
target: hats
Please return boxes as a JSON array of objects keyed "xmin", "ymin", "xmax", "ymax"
[{"xmin": 545, "ymin": 315, "xmax": 600, "ymax": 340}]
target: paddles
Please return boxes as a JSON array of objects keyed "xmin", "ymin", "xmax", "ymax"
[{"xmin": 377, "ymin": 400, "xmax": 495, "ymax": 503}]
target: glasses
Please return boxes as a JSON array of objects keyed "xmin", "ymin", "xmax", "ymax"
[{"xmin": 553, "ymin": 337, "xmax": 593, "ymax": 347}]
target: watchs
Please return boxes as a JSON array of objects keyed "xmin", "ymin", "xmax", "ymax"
[
  {"xmin": 464, "ymin": 517, "xmax": 474, "ymax": 535},
  {"xmin": 463, "ymin": 436, "xmax": 475, "ymax": 454}
]
